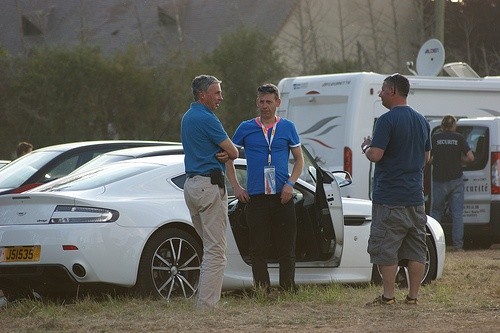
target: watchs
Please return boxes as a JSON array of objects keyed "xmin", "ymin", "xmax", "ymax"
[{"xmin": 362, "ymin": 144, "xmax": 369, "ymax": 153}]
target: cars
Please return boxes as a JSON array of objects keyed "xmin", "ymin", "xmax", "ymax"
[
  {"xmin": 0, "ymin": 154, "xmax": 446, "ymax": 304},
  {"xmin": 0, "ymin": 139, "xmax": 183, "ymax": 190},
  {"xmin": 64, "ymin": 145, "xmax": 295, "ymax": 179}
]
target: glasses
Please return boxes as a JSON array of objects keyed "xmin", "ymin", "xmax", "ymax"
[{"xmin": 391, "ymin": 73, "xmax": 400, "ymax": 95}]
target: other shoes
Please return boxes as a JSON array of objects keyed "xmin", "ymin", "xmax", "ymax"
[
  {"xmin": 366, "ymin": 294, "xmax": 396, "ymax": 308},
  {"xmin": 396, "ymin": 296, "xmax": 419, "ymax": 306}
]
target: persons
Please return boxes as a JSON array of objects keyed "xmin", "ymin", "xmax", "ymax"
[
  {"xmin": 180, "ymin": 75, "xmax": 239, "ymax": 310},
  {"xmin": 225, "ymin": 83, "xmax": 304, "ymax": 296},
  {"xmin": 427, "ymin": 115, "xmax": 474, "ymax": 252},
  {"xmin": 361, "ymin": 74, "xmax": 432, "ymax": 309},
  {"xmin": 16, "ymin": 142, "xmax": 33, "ymax": 158}
]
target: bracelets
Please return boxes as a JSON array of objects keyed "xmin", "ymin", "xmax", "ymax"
[{"xmin": 287, "ymin": 180, "xmax": 295, "ymax": 187}]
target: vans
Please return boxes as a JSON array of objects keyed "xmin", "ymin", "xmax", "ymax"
[{"xmin": 426, "ymin": 115, "xmax": 500, "ymax": 247}]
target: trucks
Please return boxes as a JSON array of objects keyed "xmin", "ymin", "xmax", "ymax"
[{"xmin": 275, "ymin": 61, "xmax": 500, "ymax": 203}]
